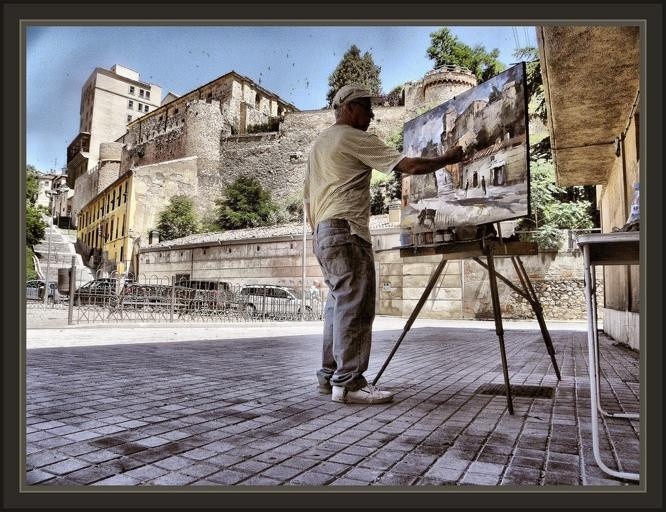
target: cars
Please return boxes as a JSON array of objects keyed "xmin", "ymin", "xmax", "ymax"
[
  {"xmin": 26, "ymin": 280, "xmax": 68, "ymax": 304},
  {"xmin": 73, "ymin": 278, "xmax": 237, "ymax": 316}
]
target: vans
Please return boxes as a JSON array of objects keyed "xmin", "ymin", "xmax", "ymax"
[{"xmin": 240, "ymin": 285, "xmax": 324, "ymax": 321}]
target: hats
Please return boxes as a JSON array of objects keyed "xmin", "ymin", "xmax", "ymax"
[{"xmin": 332, "ymin": 85, "xmax": 383, "ymax": 106}]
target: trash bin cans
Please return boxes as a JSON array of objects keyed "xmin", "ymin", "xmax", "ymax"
[{"xmin": 57, "ymin": 268, "xmax": 77, "ymax": 293}]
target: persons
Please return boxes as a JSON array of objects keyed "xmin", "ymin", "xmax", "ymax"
[
  {"xmin": 465, "ymin": 179, "xmax": 469, "ymax": 195},
  {"xmin": 482, "ymin": 176, "xmax": 486, "ymax": 194},
  {"xmin": 303, "ymin": 86, "xmax": 469, "ymax": 404}
]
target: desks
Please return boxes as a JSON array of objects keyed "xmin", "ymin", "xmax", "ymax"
[{"xmin": 577, "ymin": 232, "xmax": 644, "ymax": 483}]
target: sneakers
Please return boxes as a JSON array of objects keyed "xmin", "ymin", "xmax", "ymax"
[{"xmin": 317, "ymin": 382, "xmax": 394, "ymax": 403}]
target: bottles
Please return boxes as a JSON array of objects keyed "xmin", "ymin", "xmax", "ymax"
[{"xmin": 630, "ymin": 182, "xmax": 640, "ymax": 221}]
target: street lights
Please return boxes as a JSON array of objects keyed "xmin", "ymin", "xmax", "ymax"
[
  {"xmin": 44, "ymin": 175, "xmax": 69, "ymax": 304},
  {"xmin": 289, "ymin": 151, "xmax": 307, "ymax": 321}
]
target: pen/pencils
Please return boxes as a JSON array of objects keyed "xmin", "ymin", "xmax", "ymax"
[{"xmin": 456, "ymin": 140, "xmax": 460, "ymax": 147}]
